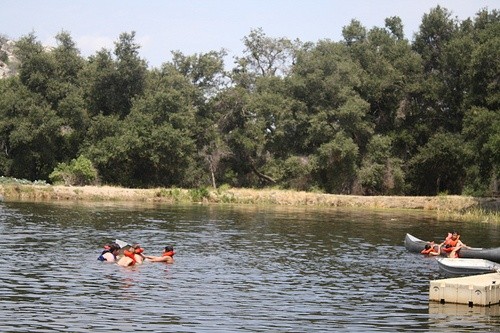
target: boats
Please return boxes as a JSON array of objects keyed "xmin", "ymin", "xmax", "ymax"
[
  {"xmin": 435, "ymin": 255, "xmax": 498, "ymax": 278},
  {"xmin": 404, "ymin": 233, "xmax": 500, "ymax": 262}
]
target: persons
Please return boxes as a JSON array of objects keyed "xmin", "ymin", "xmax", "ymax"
[
  {"xmin": 97, "ymin": 243, "xmax": 175, "ymax": 267},
  {"xmin": 421, "ymin": 231, "xmax": 466, "ymax": 258}
]
